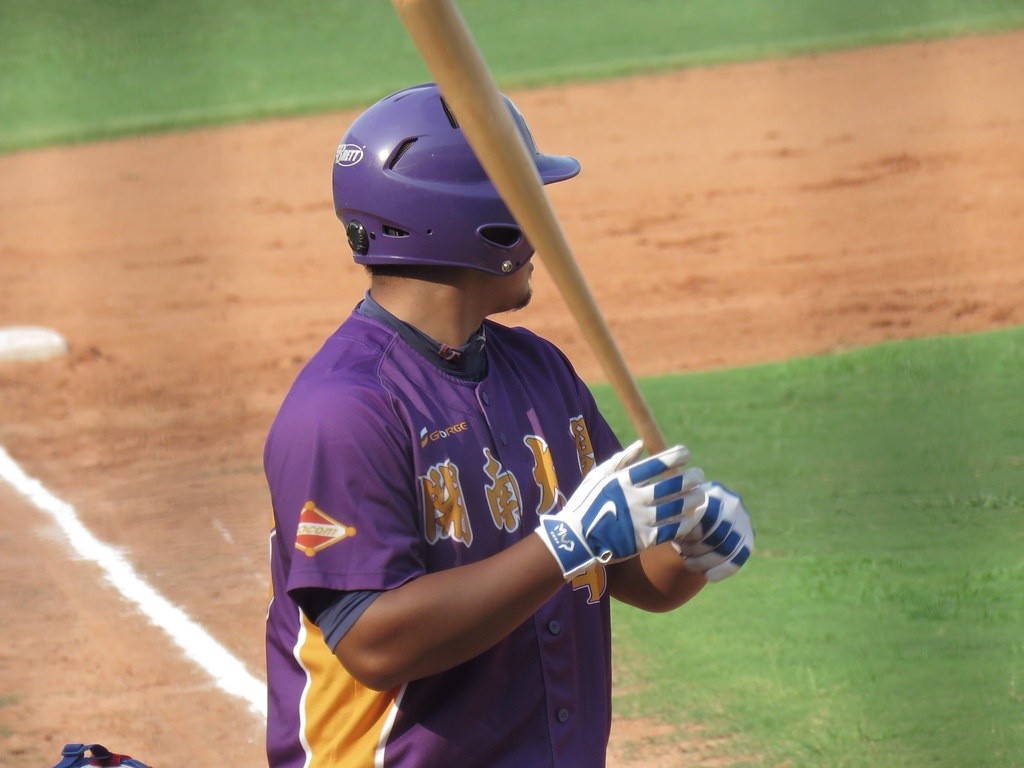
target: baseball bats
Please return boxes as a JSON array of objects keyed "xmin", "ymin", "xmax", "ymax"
[{"xmin": 381, "ymin": 0, "xmax": 705, "ymax": 543}]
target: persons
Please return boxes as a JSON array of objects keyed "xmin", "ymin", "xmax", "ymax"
[{"xmin": 264, "ymin": 81, "xmax": 755, "ymax": 768}]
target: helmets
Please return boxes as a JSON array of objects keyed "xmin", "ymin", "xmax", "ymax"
[{"xmin": 332, "ymin": 84, "xmax": 581, "ymax": 276}]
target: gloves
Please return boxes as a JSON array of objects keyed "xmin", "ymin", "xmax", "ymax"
[
  {"xmin": 670, "ymin": 483, "xmax": 755, "ymax": 582},
  {"xmin": 535, "ymin": 441, "xmax": 706, "ymax": 584}
]
